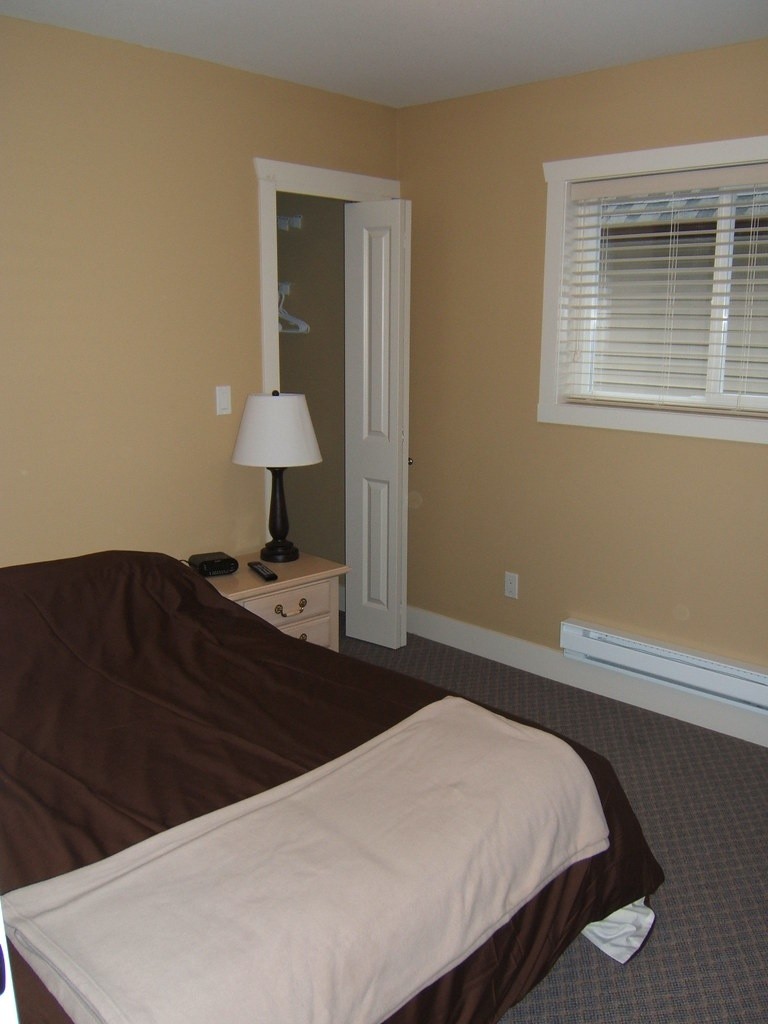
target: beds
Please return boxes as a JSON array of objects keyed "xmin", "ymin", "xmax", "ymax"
[{"xmin": 0, "ymin": 551, "xmax": 666, "ymax": 1024}]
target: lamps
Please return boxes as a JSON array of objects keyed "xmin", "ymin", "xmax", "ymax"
[{"xmin": 232, "ymin": 390, "xmax": 323, "ymax": 564}]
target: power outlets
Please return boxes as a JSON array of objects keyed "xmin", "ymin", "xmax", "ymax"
[{"xmin": 504, "ymin": 571, "xmax": 519, "ymax": 599}]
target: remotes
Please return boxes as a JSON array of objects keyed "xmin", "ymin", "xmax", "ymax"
[{"xmin": 247, "ymin": 561, "xmax": 279, "ymax": 581}]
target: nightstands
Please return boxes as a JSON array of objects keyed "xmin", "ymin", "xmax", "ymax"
[{"xmin": 206, "ymin": 550, "xmax": 354, "ymax": 654}]
